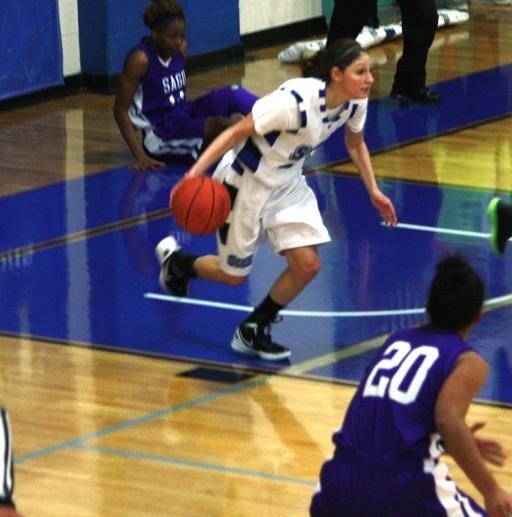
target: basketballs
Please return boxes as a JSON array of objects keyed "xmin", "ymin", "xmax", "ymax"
[{"xmin": 171, "ymin": 176, "xmax": 231, "ymax": 236}]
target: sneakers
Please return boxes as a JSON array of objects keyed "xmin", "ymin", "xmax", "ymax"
[
  {"xmin": 385, "ymin": 81, "xmax": 442, "ymax": 107},
  {"xmin": 153, "ymin": 235, "xmax": 188, "ymax": 298},
  {"xmin": 229, "ymin": 315, "xmax": 292, "ymax": 362}
]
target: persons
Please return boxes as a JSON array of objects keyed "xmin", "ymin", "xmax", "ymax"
[
  {"xmin": 153, "ymin": 38, "xmax": 398, "ymax": 363},
  {"xmin": 307, "ymin": 253, "xmax": 512, "ymax": 517},
  {"xmin": 110, "ymin": 1, "xmax": 259, "ymax": 173},
  {"xmin": 1, "ymin": 405, "xmax": 23, "ymax": 517},
  {"xmin": 391, "ymin": 0, "xmax": 441, "ymax": 107},
  {"xmin": 322, "ymin": 0, "xmax": 378, "ymax": 47}
]
